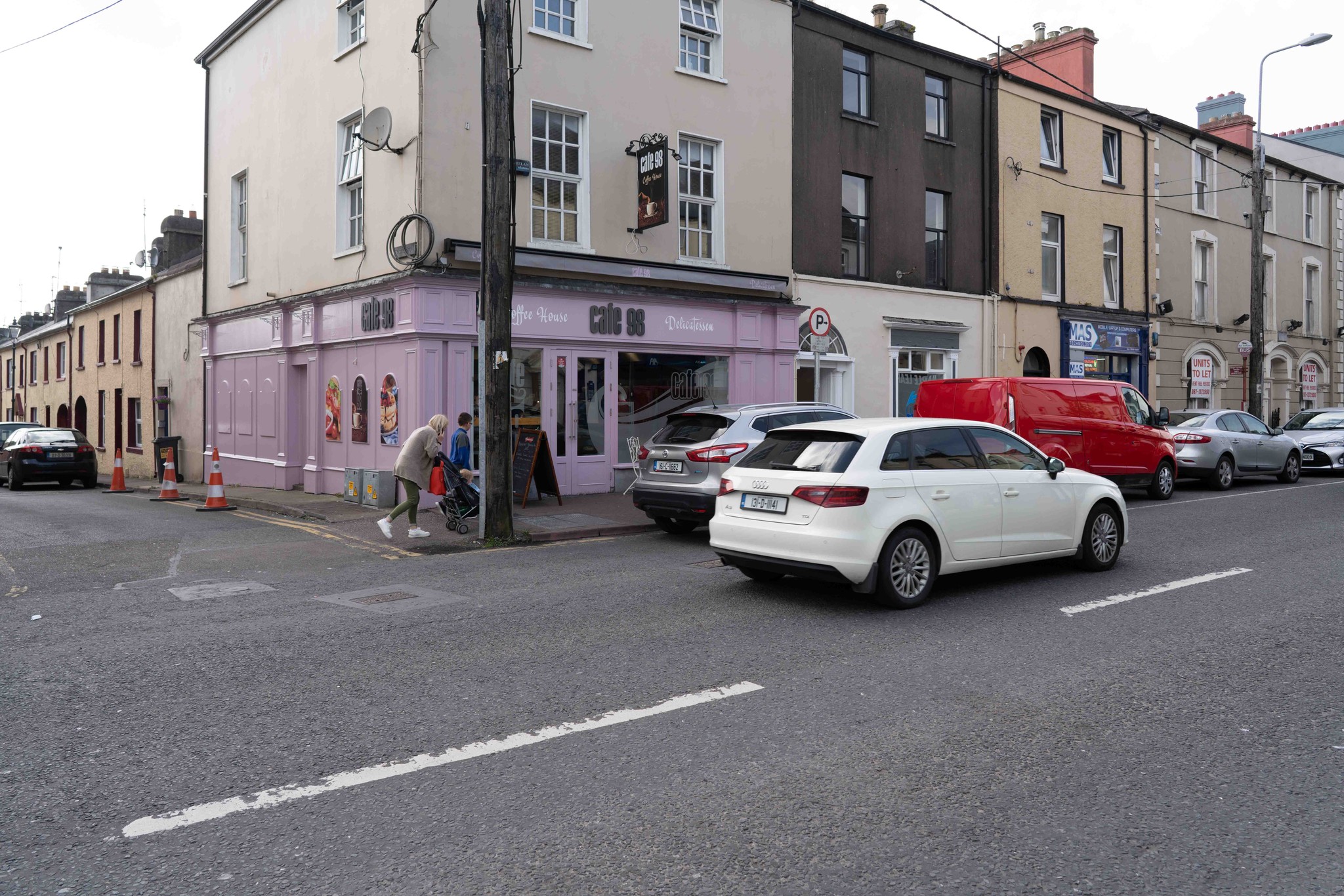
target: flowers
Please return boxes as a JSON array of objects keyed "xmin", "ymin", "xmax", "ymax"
[{"xmin": 151, "ymin": 394, "xmax": 171, "ymax": 403}]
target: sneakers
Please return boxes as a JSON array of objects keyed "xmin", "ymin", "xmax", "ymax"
[
  {"xmin": 435, "ymin": 501, "xmax": 447, "ymax": 518},
  {"xmin": 377, "ymin": 519, "xmax": 392, "ymax": 539},
  {"xmin": 408, "ymin": 527, "xmax": 430, "ymax": 538}
]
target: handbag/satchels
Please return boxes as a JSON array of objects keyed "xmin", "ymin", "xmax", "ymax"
[{"xmin": 427, "ymin": 460, "xmax": 446, "ymax": 495}]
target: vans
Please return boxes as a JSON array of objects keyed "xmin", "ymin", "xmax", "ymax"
[{"xmin": 910, "ymin": 375, "xmax": 1179, "ymax": 501}]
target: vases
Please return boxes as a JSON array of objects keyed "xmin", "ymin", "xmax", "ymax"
[{"xmin": 157, "ymin": 402, "xmax": 169, "ymax": 410}]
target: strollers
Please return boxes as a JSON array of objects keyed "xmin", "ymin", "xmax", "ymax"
[{"xmin": 433, "ymin": 451, "xmax": 480, "ymax": 535}]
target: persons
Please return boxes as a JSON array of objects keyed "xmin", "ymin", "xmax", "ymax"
[
  {"xmin": 435, "ymin": 412, "xmax": 477, "ymax": 520},
  {"xmin": 459, "ymin": 468, "xmax": 480, "ymax": 494},
  {"xmin": 377, "ymin": 414, "xmax": 450, "ymax": 538}
]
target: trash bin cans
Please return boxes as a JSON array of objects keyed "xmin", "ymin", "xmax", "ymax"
[{"xmin": 151, "ymin": 436, "xmax": 183, "ymax": 484}]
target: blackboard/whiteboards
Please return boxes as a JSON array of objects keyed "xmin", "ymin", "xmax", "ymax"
[{"xmin": 512, "ymin": 428, "xmax": 561, "ymax": 498}]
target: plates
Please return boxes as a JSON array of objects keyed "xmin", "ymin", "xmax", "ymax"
[{"xmin": 352, "ymin": 426, "xmax": 363, "ymax": 429}]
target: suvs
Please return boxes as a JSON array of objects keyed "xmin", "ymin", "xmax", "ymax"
[{"xmin": 631, "ymin": 399, "xmax": 931, "ymax": 538}]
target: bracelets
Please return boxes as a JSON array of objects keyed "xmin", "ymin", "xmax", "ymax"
[{"xmin": 438, "ymin": 441, "xmax": 441, "ymax": 445}]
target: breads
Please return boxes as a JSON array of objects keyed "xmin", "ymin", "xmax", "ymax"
[{"xmin": 380, "ymin": 392, "xmax": 397, "ymax": 432}]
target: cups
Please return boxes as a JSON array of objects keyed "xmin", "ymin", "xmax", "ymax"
[
  {"xmin": 386, "ymin": 382, "xmax": 397, "ymax": 401},
  {"xmin": 381, "ymin": 425, "xmax": 398, "ymax": 445},
  {"xmin": 353, "ymin": 413, "xmax": 362, "ymax": 427},
  {"xmin": 326, "ymin": 410, "xmax": 333, "ymax": 434}
]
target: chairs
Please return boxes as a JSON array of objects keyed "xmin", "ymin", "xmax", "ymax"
[{"xmin": 623, "ymin": 437, "xmax": 640, "ymax": 495}]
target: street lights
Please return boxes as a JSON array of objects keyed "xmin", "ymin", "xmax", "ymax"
[
  {"xmin": 1248, "ymin": 32, "xmax": 1335, "ymax": 421},
  {"xmin": 7, "ymin": 316, "xmax": 22, "ymax": 421}
]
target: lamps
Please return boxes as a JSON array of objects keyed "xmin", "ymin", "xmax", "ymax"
[
  {"xmin": 630, "ymin": 141, "xmax": 682, "ymax": 161},
  {"xmin": 1338, "ymin": 326, "xmax": 1344, "ymax": 336},
  {"xmin": 1156, "ymin": 299, "xmax": 1173, "ymax": 315},
  {"xmin": 1205, "ymin": 325, "xmax": 1223, "ymax": 333},
  {"xmin": 1278, "ymin": 319, "xmax": 1302, "ymax": 332},
  {"xmin": 1313, "ymin": 338, "xmax": 1328, "ymax": 345},
  {"xmin": 1234, "ymin": 314, "xmax": 1250, "ymax": 324}
]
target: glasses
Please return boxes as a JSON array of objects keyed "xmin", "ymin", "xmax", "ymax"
[{"xmin": 465, "ymin": 423, "xmax": 472, "ymax": 426}]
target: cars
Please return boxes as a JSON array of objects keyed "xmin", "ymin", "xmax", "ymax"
[
  {"xmin": 704, "ymin": 414, "xmax": 1130, "ymax": 610},
  {"xmin": 0, "ymin": 420, "xmax": 98, "ymax": 491},
  {"xmin": 1146, "ymin": 408, "xmax": 1304, "ymax": 492},
  {"xmin": 1272, "ymin": 406, "xmax": 1344, "ymax": 478}
]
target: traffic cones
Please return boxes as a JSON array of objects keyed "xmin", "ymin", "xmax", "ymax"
[
  {"xmin": 195, "ymin": 447, "xmax": 238, "ymax": 512},
  {"xmin": 101, "ymin": 448, "xmax": 135, "ymax": 493},
  {"xmin": 148, "ymin": 447, "xmax": 189, "ymax": 502}
]
